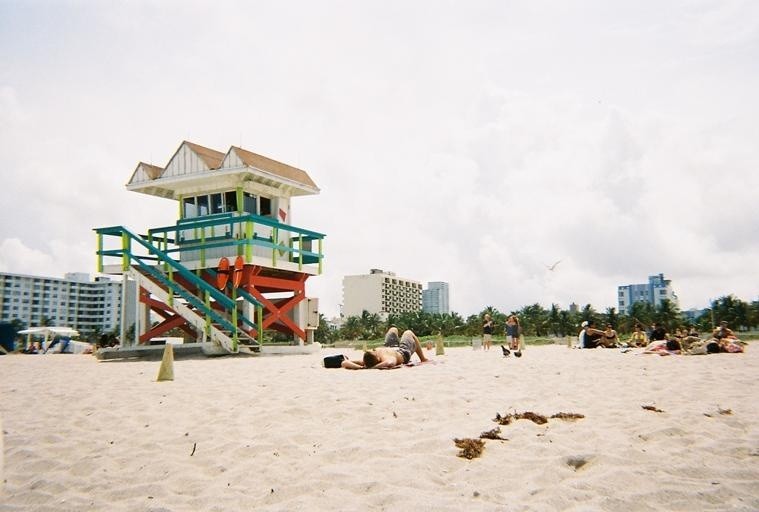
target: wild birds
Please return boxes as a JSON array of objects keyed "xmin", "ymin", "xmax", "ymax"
[
  {"xmin": 541, "ymin": 258, "xmax": 563, "ymax": 270},
  {"xmin": 513, "ymin": 348, "xmax": 523, "ymax": 358},
  {"xmin": 500, "ymin": 345, "xmax": 510, "ymax": 357}
]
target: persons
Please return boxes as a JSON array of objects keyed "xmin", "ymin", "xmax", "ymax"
[
  {"xmin": 512, "ymin": 316, "xmax": 521, "ymax": 350},
  {"xmin": 583, "ymin": 320, "xmax": 622, "ymax": 348},
  {"xmin": 40, "ymin": 336, "xmax": 46, "ymax": 351},
  {"xmin": 578, "ymin": 321, "xmax": 588, "ymax": 348},
  {"xmin": 650, "ymin": 320, "xmax": 734, "ymax": 343},
  {"xmin": 482, "ymin": 313, "xmax": 494, "ymax": 349},
  {"xmin": 504, "ymin": 314, "xmax": 513, "ymax": 349},
  {"xmin": 426, "ymin": 337, "xmax": 433, "ymax": 350},
  {"xmin": 341, "ymin": 326, "xmax": 429, "ymax": 370},
  {"xmin": 623, "ymin": 322, "xmax": 648, "ymax": 348},
  {"xmin": 601, "ymin": 323, "xmax": 618, "ymax": 348},
  {"xmin": 28, "ymin": 343, "xmax": 36, "ymax": 353}
]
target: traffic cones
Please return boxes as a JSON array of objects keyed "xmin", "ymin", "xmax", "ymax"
[
  {"xmin": 157, "ymin": 343, "xmax": 175, "ymax": 381},
  {"xmin": 436, "ymin": 334, "xmax": 444, "ymax": 355}
]
